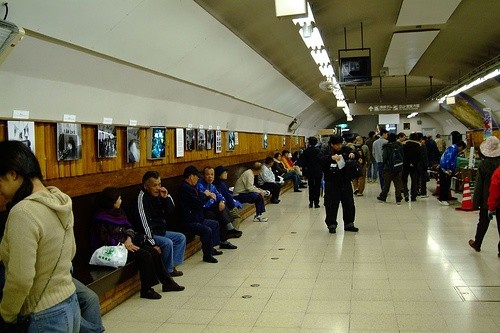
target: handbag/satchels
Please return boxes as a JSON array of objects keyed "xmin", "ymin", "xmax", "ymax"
[
  {"xmin": 336, "ymin": 157, "xmax": 363, "ymax": 180},
  {"xmin": 89, "ymin": 242, "xmax": 128, "ymax": 268}
]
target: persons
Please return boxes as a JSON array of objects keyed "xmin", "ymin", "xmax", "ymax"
[
  {"xmin": 469, "ymin": 136, "xmax": 500, "ymax": 257},
  {"xmin": 0, "ymin": 140, "xmax": 105, "ymax": 333},
  {"xmin": 295, "ymin": 128, "xmax": 467, "ymax": 232},
  {"xmin": 229, "ymin": 137, "xmax": 235, "ymax": 150},
  {"xmin": 233, "ymin": 149, "xmax": 307, "ymax": 222},
  {"xmin": 198, "ymin": 136, "xmax": 204, "ymax": 144},
  {"xmin": 99, "ymin": 135, "xmax": 117, "ymax": 156},
  {"xmin": 348, "ymin": 67, "xmax": 358, "ymax": 76},
  {"xmin": 95, "ymin": 171, "xmax": 186, "ymax": 299},
  {"xmin": 211, "ymin": 135, "xmax": 214, "ymax": 148},
  {"xmin": 172, "ymin": 166, "xmax": 242, "ymax": 263},
  {"xmin": 187, "ymin": 136, "xmax": 194, "ymax": 149}
]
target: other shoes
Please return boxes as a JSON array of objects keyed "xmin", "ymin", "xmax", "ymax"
[
  {"xmin": 227, "ymin": 230, "xmax": 241, "ymax": 238},
  {"xmin": 271, "ymin": 200, "xmax": 279, "ymax": 204},
  {"xmin": 264, "ymin": 190, "xmax": 271, "ymax": 196},
  {"xmin": 498, "ymin": 253, "xmax": 500, "ymax": 257},
  {"xmin": 219, "ymin": 242, "xmax": 237, "ymax": 249},
  {"xmin": 212, "ymin": 249, "xmax": 223, "ymax": 255},
  {"xmin": 299, "ymin": 186, "xmax": 307, "ymax": 188},
  {"xmin": 309, "ymin": 204, "xmax": 313, "ymax": 207},
  {"xmin": 300, "ymin": 182, "xmax": 307, "ymax": 185},
  {"xmin": 140, "ymin": 288, "xmax": 161, "ymax": 299},
  {"xmin": 162, "ymin": 283, "xmax": 185, "ymax": 291},
  {"xmin": 171, "ymin": 268, "xmax": 183, "ymax": 276},
  {"xmin": 203, "ymin": 256, "xmax": 218, "ymax": 263},
  {"xmin": 277, "ymin": 200, "xmax": 280, "ymax": 202},
  {"xmin": 232, "ymin": 228, "xmax": 242, "ymax": 235},
  {"xmin": 469, "ymin": 238, "xmax": 480, "ymax": 251},
  {"xmin": 253, "ymin": 215, "xmax": 269, "ymax": 222},
  {"xmin": 353, "ymin": 179, "xmax": 458, "ymax": 204},
  {"xmin": 329, "ymin": 228, "xmax": 336, "ymax": 233},
  {"xmin": 344, "ymin": 226, "xmax": 358, "ymax": 232},
  {"xmin": 294, "ymin": 190, "xmax": 302, "ymax": 192},
  {"xmin": 315, "ymin": 204, "xmax": 320, "ymax": 208}
]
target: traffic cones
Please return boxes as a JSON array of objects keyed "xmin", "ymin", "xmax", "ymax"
[{"xmin": 455, "ymin": 176, "xmax": 480, "ymax": 211}]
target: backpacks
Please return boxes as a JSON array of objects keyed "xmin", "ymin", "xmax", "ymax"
[{"xmin": 354, "ymin": 144, "xmax": 366, "ymax": 161}]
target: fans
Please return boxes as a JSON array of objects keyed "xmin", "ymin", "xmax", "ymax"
[{"xmin": 320, "ymin": 72, "xmax": 342, "ymax": 93}]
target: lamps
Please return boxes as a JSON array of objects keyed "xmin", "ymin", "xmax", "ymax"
[
  {"xmin": 437, "ymin": 63, "xmax": 500, "ymax": 103},
  {"xmin": 291, "ymin": 1, "xmax": 351, "ymax": 116}
]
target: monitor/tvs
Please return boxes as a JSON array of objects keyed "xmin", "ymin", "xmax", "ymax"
[
  {"xmin": 339, "ymin": 56, "xmax": 372, "ymax": 87},
  {"xmin": 226, "ymin": 132, "xmax": 235, "ymax": 152},
  {"xmin": 336, "ymin": 124, "xmax": 351, "ymax": 135},
  {"xmin": 377, "ymin": 123, "xmax": 397, "ymax": 134},
  {"xmin": 146, "ymin": 127, "xmax": 167, "ymax": 160}
]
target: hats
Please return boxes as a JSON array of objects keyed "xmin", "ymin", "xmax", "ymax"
[
  {"xmin": 214, "ymin": 166, "xmax": 229, "ymax": 179},
  {"xmin": 480, "ymin": 136, "xmax": 500, "ymax": 157}
]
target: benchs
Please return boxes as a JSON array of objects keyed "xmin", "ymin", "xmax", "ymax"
[{"xmin": 83, "ymin": 178, "xmax": 293, "ymax": 317}]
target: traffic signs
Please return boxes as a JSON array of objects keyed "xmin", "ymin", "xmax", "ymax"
[{"xmin": 349, "ymin": 100, "xmax": 440, "ymax": 115}]
